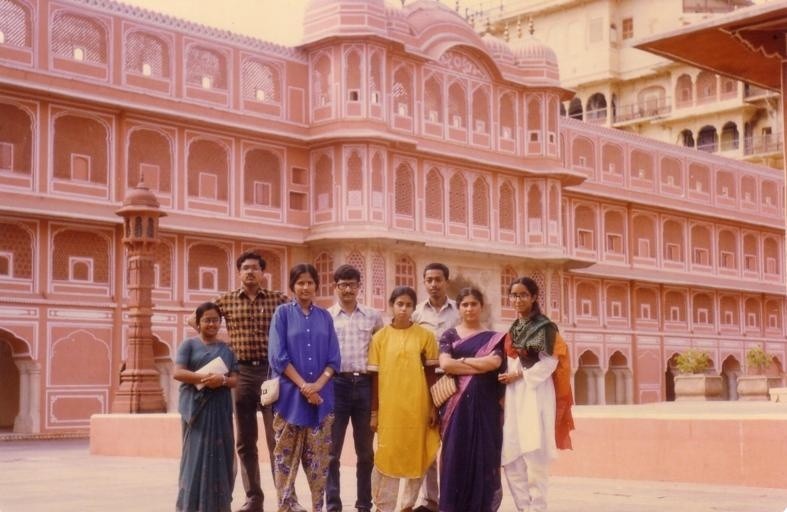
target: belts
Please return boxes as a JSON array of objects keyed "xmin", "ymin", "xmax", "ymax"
[
  {"xmin": 238, "ymin": 359, "xmax": 268, "ymax": 367},
  {"xmin": 336, "ymin": 372, "xmax": 371, "ymax": 378}
]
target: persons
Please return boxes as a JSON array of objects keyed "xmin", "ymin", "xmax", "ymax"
[
  {"xmin": 268, "ymin": 263, "xmax": 341, "ymax": 512},
  {"xmin": 437, "ymin": 287, "xmax": 508, "ymax": 512},
  {"xmin": 321, "ymin": 265, "xmax": 384, "ymax": 512},
  {"xmin": 366, "ymin": 286, "xmax": 437, "ymax": 512},
  {"xmin": 498, "ymin": 277, "xmax": 576, "ymax": 512},
  {"xmin": 412, "ymin": 262, "xmax": 464, "ymax": 512},
  {"xmin": 187, "ymin": 252, "xmax": 293, "ymax": 512},
  {"xmin": 172, "ymin": 302, "xmax": 240, "ymax": 512}
]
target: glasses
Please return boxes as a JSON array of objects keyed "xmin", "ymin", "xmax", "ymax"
[{"xmin": 335, "ymin": 282, "xmax": 360, "ymax": 290}]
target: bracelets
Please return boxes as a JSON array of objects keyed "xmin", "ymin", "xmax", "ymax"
[
  {"xmin": 370, "ymin": 414, "xmax": 377, "ymax": 416},
  {"xmin": 323, "ymin": 371, "xmax": 333, "ymax": 378},
  {"xmin": 460, "ymin": 357, "xmax": 465, "ymax": 363},
  {"xmin": 370, "ymin": 411, "xmax": 378, "ymax": 414},
  {"xmin": 515, "ymin": 370, "xmax": 521, "ymax": 380},
  {"xmin": 223, "ymin": 375, "xmax": 228, "ymax": 387}
]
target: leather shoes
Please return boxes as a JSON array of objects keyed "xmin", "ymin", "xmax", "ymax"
[{"xmin": 238, "ymin": 502, "xmax": 263, "ymax": 512}]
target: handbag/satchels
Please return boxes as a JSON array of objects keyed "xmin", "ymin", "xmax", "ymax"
[
  {"xmin": 431, "ymin": 373, "xmax": 458, "ymax": 408},
  {"xmin": 261, "ymin": 364, "xmax": 280, "ymax": 405},
  {"xmin": 192, "ymin": 357, "xmax": 229, "ymax": 390}
]
